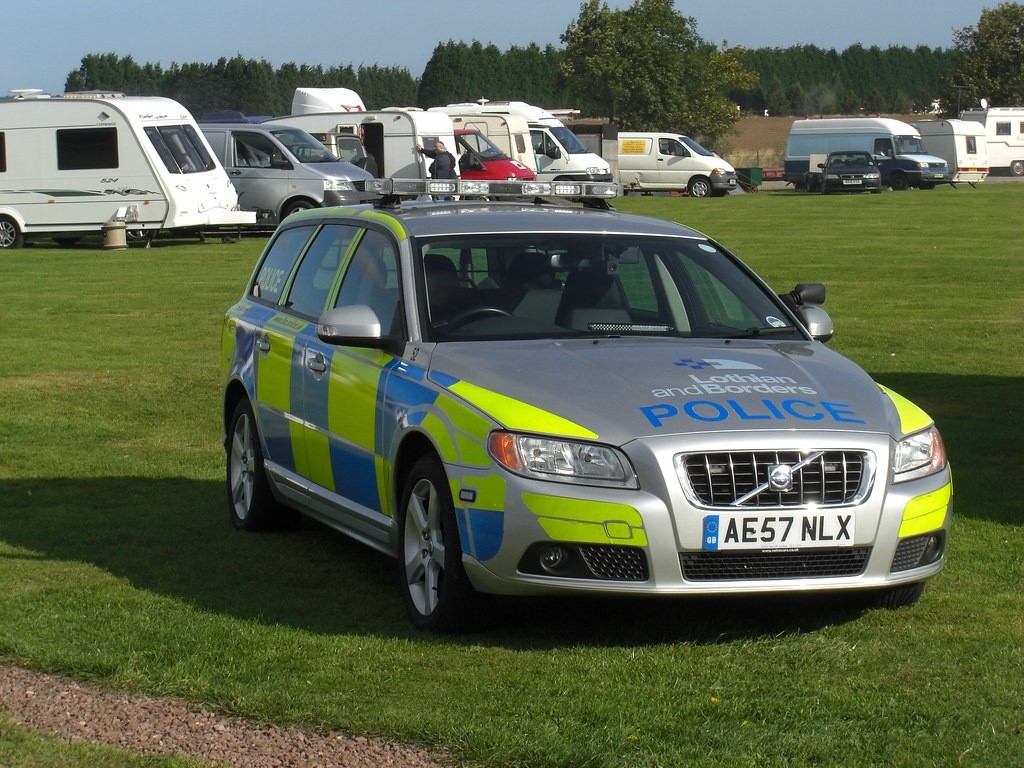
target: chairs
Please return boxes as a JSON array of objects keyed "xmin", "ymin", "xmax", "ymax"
[
  {"xmin": 855, "ymin": 157, "xmax": 867, "ymax": 168},
  {"xmin": 833, "ymin": 158, "xmax": 845, "ymax": 168},
  {"xmin": 338, "ymin": 255, "xmax": 391, "ymax": 320},
  {"xmin": 390, "ymin": 253, "xmax": 487, "ymax": 343},
  {"xmin": 501, "ymin": 251, "xmax": 563, "ymax": 303},
  {"xmin": 557, "ymin": 265, "xmax": 633, "ymax": 332}
]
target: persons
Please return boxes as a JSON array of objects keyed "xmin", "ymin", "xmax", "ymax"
[{"xmin": 415, "ymin": 140, "xmax": 457, "ymax": 201}]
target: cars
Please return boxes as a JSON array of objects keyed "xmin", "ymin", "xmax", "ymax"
[
  {"xmin": 817, "ymin": 150, "xmax": 883, "ymax": 194},
  {"xmin": 220, "ymin": 177, "xmax": 954, "ymax": 635}
]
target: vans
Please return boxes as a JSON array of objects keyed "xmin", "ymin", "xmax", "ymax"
[
  {"xmin": 124, "ymin": 121, "xmax": 383, "ymax": 241},
  {"xmin": 276, "ymin": 132, "xmax": 366, "ymax": 173},
  {"xmin": 452, "ymin": 129, "xmax": 536, "ymax": 184},
  {"xmin": 427, "ymin": 101, "xmax": 612, "ymax": 199},
  {"xmin": 784, "ymin": 116, "xmax": 949, "ymax": 190},
  {"xmin": 958, "ymin": 107, "xmax": 1024, "ymax": 177},
  {"xmin": 0, "ymin": 89, "xmax": 259, "ymax": 250},
  {"xmin": 904, "ymin": 120, "xmax": 989, "ymax": 190},
  {"xmin": 252, "ymin": 88, "xmax": 460, "ymax": 180},
  {"xmin": 616, "ymin": 132, "xmax": 736, "ymax": 197}
]
